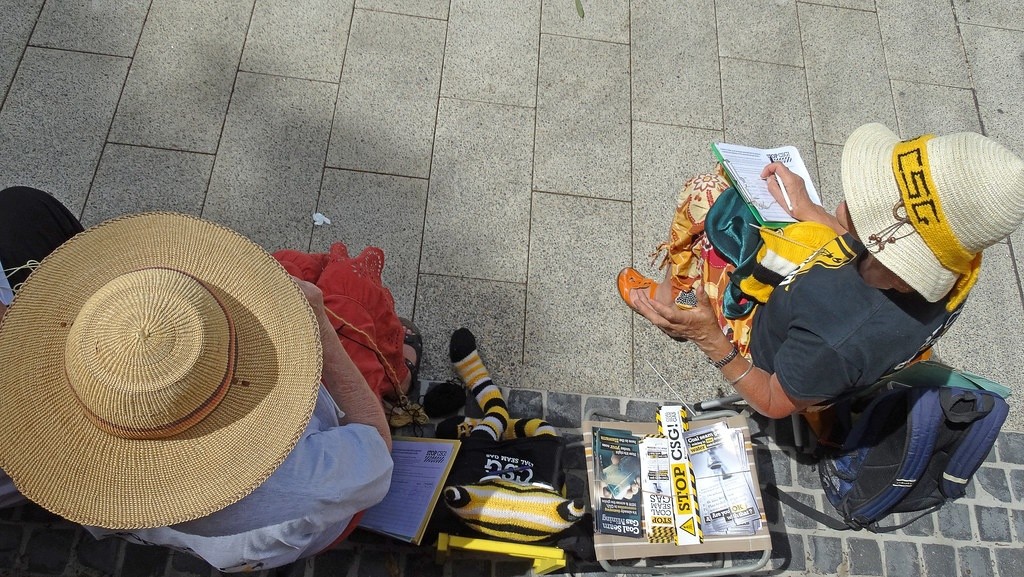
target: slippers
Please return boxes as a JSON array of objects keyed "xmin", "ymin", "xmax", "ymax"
[{"xmin": 387, "ymin": 316, "xmax": 422, "ymax": 401}]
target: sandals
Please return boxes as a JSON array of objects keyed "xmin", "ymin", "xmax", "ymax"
[{"xmin": 617, "ymin": 268, "xmax": 659, "ymax": 315}]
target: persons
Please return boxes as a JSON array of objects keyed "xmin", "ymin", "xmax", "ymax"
[
  {"xmin": 1, "ymin": 186, "xmax": 422, "ymax": 573},
  {"xmin": 618, "ymin": 123, "xmax": 1024, "ymax": 420}
]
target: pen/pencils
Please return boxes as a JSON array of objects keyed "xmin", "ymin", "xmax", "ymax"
[
  {"xmin": 769, "ymin": 156, "xmax": 793, "ymax": 212},
  {"xmin": 488, "ymin": 466, "xmax": 534, "ymax": 476}
]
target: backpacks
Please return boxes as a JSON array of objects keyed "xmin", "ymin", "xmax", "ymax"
[{"xmin": 765, "ymin": 361, "xmax": 1013, "ymax": 533}]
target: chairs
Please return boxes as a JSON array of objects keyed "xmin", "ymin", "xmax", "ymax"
[{"xmin": 694, "ymin": 356, "xmax": 1012, "ymax": 536}]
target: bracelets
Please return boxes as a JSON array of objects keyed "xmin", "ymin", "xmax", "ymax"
[
  {"xmin": 706, "ymin": 343, "xmax": 737, "ymax": 368},
  {"xmin": 729, "ymin": 363, "xmax": 753, "ymax": 386}
]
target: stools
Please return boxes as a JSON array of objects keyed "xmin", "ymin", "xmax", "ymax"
[{"xmin": 582, "ymin": 406, "xmax": 775, "ymax": 577}]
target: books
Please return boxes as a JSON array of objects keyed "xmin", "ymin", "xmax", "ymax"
[{"xmin": 712, "ymin": 142, "xmax": 821, "ymax": 228}]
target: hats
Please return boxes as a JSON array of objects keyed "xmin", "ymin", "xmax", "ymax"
[
  {"xmin": 0, "ymin": 211, "xmax": 323, "ymax": 530},
  {"xmin": 841, "ymin": 122, "xmax": 1024, "ymax": 302}
]
